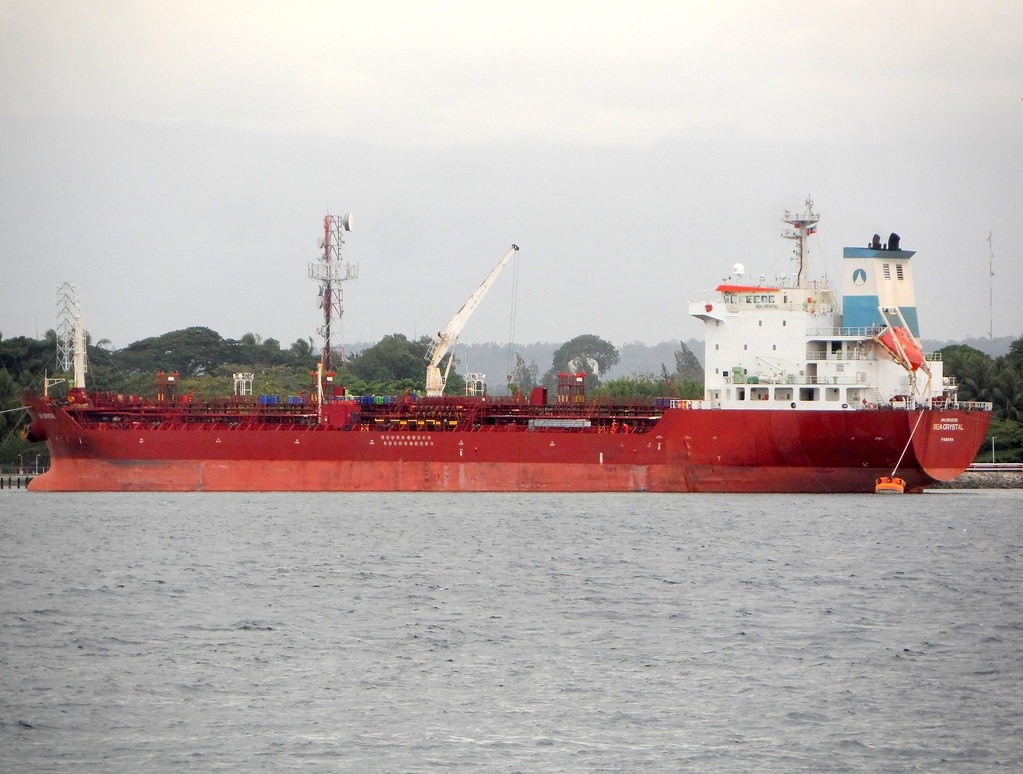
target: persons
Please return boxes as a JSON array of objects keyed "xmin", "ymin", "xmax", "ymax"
[
  {"xmin": 623, "ymin": 423, "xmax": 628, "ymax": 434},
  {"xmin": 611, "ymin": 419, "xmax": 616, "ymax": 432}
]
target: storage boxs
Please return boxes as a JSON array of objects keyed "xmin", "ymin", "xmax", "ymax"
[{"xmin": 258, "ymin": 393, "xmax": 418, "ymax": 405}]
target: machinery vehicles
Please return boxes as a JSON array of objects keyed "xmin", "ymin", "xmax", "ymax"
[{"xmin": 424, "ymin": 242, "xmax": 521, "ymax": 396}]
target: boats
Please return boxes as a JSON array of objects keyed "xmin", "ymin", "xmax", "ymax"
[{"xmin": 23, "ymin": 192, "xmax": 996, "ymax": 495}]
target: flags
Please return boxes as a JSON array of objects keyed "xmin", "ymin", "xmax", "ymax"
[{"xmin": 808, "ymin": 225, "xmax": 816, "ymax": 235}]
